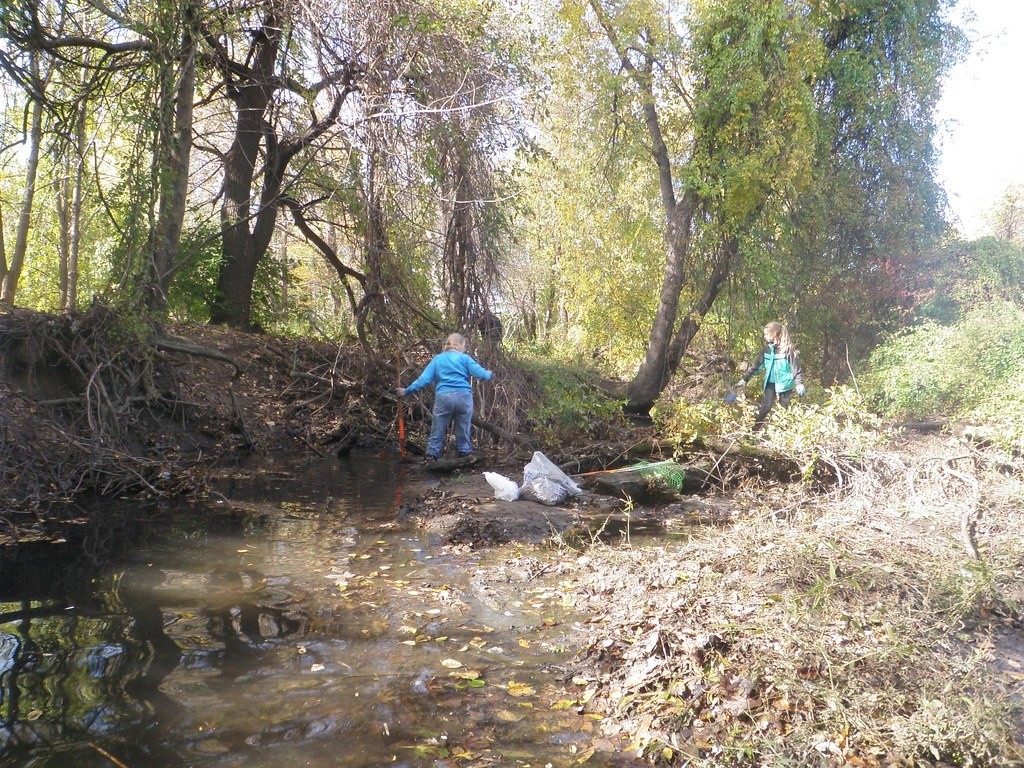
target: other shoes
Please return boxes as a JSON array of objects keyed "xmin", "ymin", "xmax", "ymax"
[
  {"xmin": 424, "ymin": 454, "xmax": 437, "ymax": 462},
  {"xmin": 748, "ymin": 420, "xmax": 765, "ymax": 432},
  {"xmin": 459, "ymin": 452, "xmax": 472, "ymax": 457}
]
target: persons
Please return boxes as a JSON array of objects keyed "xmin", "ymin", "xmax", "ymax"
[
  {"xmin": 735, "ymin": 322, "xmax": 806, "ymax": 431},
  {"xmin": 395, "ymin": 333, "xmax": 494, "ymax": 461}
]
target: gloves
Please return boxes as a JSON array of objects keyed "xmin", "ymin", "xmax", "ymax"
[
  {"xmin": 735, "ymin": 379, "xmax": 746, "ymax": 388},
  {"xmin": 796, "ymin": 384, "xmax": 805, "ymax": 396}
]
